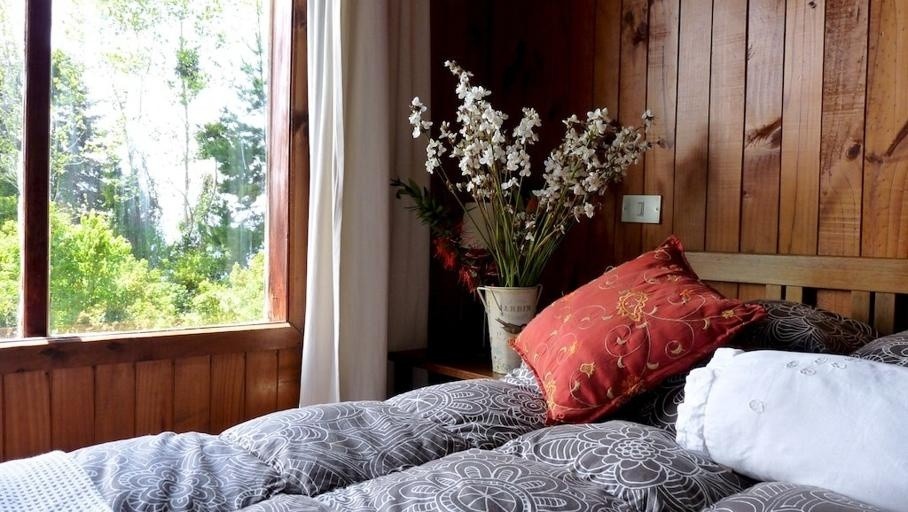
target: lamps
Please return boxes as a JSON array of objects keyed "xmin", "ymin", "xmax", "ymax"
[{"xmin": 461, "ymin": 199, "xmax": 511, "ymax": 364}]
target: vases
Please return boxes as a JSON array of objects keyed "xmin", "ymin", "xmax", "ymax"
[{"xmin": 475, "ymin": 282, "xmax": 543, "ymax": 377}]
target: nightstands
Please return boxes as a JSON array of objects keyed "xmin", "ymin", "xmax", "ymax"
[{"xmin": 382, "ymin": 344, "xmax": 504, "ymax": 398}]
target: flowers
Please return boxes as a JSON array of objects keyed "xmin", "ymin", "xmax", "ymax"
[{"xmin": 383, "ymin": 51, "xmax": 664, "ymax": 303}]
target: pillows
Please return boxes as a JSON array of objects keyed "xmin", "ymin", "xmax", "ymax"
[
  {"xmin": 674, "ymin": 343, "xmax": 907, "ymax": 512},
  {"xmin": 844, "ymin": 329, "xmax": 907, "ymax": 366},
  {"xmin": 506, "ymin": 234, "xmax": 766, "ymax": 428},
  {"xmin": 710, "ymin": 298, "xmax": 883, "ymax": 360}
]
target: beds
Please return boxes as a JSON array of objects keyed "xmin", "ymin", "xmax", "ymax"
[{"xmin": 3, "ymin": 249, "xmax": 907, "ymax": 512}]
